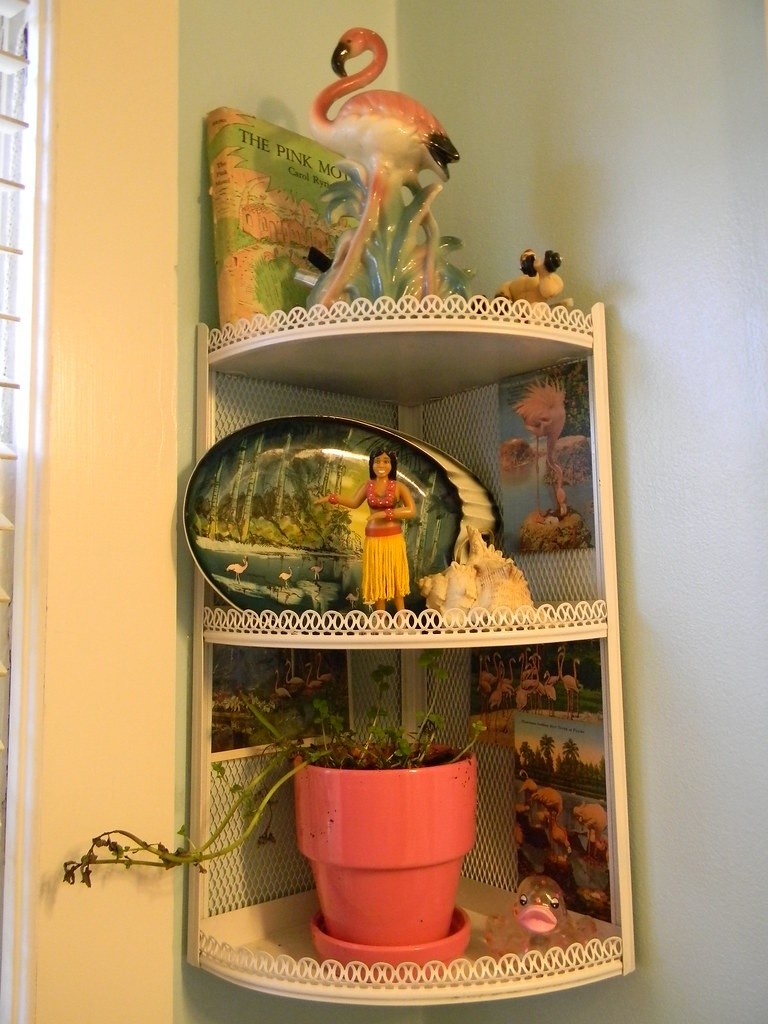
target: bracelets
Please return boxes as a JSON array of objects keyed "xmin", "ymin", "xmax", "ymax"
[
  {"xmin": 329, "ymin": 493, "xmax": 338, "ymax": 504},
  {"xmin": 385, "ymin": 508, "xmax": 394, "ymax": 522}
]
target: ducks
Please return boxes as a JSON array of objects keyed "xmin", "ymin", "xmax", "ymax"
[{"xmin": 484, "ymin": 874, "xmax": 600, "ymax": 960}]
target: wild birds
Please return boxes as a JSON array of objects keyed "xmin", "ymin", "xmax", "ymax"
[{"xmin": 307, "ymin": 25, "xmax": 462, "ymax": 318}]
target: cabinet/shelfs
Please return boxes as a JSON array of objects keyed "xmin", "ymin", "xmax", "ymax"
[{"xmin": 183, "ymin": 294, "xmax": 627, "ymax": 1006}]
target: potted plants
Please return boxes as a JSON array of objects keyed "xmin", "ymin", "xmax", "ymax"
[{"xmin": 61, "ymin": 648, "xmax": 486, "ymax": 980}]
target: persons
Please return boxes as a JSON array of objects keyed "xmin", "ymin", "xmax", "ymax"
[{"xmin": 314, "ymin": 445, "xmax": 419, "ymax": 634}]
